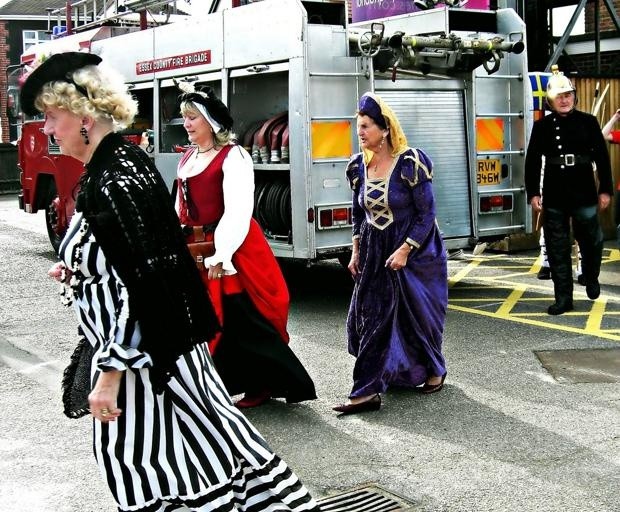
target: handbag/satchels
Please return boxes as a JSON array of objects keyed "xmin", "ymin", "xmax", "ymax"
[
  {"xmin": 187, "ymin": 242, "xmax": 215, "ymax": 272},
  {"xmin": 61, "ymin": 336, "xmax": 93, "ymax": 418}
]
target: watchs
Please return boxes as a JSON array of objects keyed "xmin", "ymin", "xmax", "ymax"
[{"xmin": 405, "ymin": 241, "xmax": 414, "ymax": 250}]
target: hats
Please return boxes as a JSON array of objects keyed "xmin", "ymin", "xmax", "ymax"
[
  {"xmin": 19, "ymin": 52, "xmax": 103, "ymax": 116},
  {"xmin": 358, "ymin": 92, "xmax": 402, "ymax": 146},
  {"xmin": 177, "ymin": 85, "xmax": 234, "ymax": 132}
]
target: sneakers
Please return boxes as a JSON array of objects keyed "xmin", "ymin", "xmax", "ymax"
[{"xmin": 537, "ymin": 266, "xmax": 600, "ymax": 315}]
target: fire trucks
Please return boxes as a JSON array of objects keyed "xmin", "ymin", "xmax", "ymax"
[{"xmin": 6, "ymin": 0, "xmax": 533, "ymax": 267}]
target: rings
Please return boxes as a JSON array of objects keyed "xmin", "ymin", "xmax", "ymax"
[
  {"xmin": 102, "ymin": 408, "xmax": 108, "ymax": 417},
  {"xmin": 218, "ymin": 274, "xmax": 222, "ymax": 278}
]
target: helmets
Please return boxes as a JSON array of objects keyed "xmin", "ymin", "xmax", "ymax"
[{"xmin": 547, "ymin": 75, "xmax": 574, "ymax": 95}]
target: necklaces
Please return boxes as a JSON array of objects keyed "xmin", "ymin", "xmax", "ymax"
[{"xmin": 374, "ymin": 153, "xmax": 382, "ymax": 172}]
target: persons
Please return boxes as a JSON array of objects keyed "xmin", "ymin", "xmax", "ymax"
[
  {"xmin": 601, "ymin": 108, "xmax": 620, "ymax": 213},
  {"xmin": 523, "ymin": 64, "xmax": 614, "ymax": 316},
  {"xmin": 171, "ymin": 85, "xmax": 318, "ymax": 408},
  {"xmin": 330, "ymin": 91, "xmax": 449, "ymax": 413},
  {"xmin": 536, "ymin": 154, "xmax": 587, "ymax": 286},
  {"xmin": 15, "ymin": 39, "xmax": 321, "ymax": 512}
]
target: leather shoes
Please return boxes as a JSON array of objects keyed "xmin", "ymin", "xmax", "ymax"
[
  {"xmin": 422, "ymin": 372, "xmax": 446, "ymax": 394},
  {"xmin": 332, "ymin": 394, "xmax": 381, "ymax": 413},
  {"xmin": 236, "ymin": 396, "xmax": 270, "ymax": 407}
]
